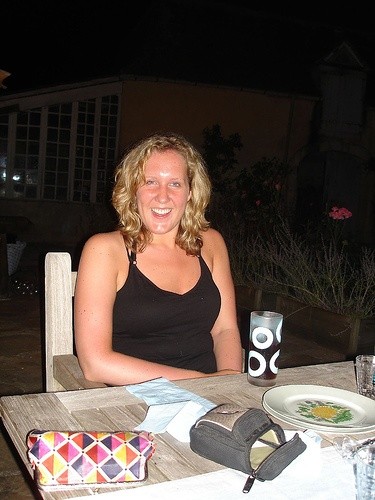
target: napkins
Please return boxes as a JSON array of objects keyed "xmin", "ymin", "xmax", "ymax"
[
  {"xmin": 126, "ymin": 376, "xmax": 218, "ymax": 413},
  {"xmin": 282, "ymin": 428, "xmax": 321, "ymax": 480},
  {"xmin": 133, "ymin": 400, "xmax": 210, "ymax": 443}
]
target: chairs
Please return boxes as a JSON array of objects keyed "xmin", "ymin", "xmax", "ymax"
[
  {"xmin": 6, "ymin": 240, "xmax": 28, "ymax": 278},
  {"xmin": 43, "ymin": 251, "xmax": 246, "ymax": 392},
  {"xmin": 0, "ymin": 232, "xmax": 9, "ymax": 290}
]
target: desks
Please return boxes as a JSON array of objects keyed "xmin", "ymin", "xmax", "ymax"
[
  {"xmin": 0, "ymin": 216, "xmax": 33, "ymax": 243},
  {"xmin": 0, "ymin": 359, "xmax": 375, "ymax": 500}
]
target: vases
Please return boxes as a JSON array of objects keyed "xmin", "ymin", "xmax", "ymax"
[
  {"xmin": 274, "ymin": 292, "xmax": 375, "ymax": 355},
  {"xmin": 233, "ymin": 285, "xmax": 278, "ymax": 311}
]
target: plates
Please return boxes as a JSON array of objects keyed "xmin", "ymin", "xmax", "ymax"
[{"xmin": 263, "ymin": 384, "xmax": 375, "ymax": 435}]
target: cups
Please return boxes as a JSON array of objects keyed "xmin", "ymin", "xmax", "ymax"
[
  {"xmin": 247, "ymin": 311, "xmax": 283, "ymax": 386},
  {"xmin": 355, "ymin": 355, "xmax": 375, "ymax": 398},
  {"xmin": 353, "ymin": 439, "xmax": 375, "ymax": 499}
]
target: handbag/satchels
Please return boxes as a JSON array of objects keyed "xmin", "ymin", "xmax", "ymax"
[
  {"xmin": 26, "ymin": 428, "xmax": 154, "ymax": 486},
  {"xmin": 189, "ymin": 401, "xmax": 307, "ymax": 494}
]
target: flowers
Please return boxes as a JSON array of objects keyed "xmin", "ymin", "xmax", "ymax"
[{"xmin": 205, "ymin": 153, "xmax": 375, "ymax": 320}]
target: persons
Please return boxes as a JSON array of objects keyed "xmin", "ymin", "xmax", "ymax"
[{"xmin": 74, "ymin": 132, "xmax": 243, "ymax": 386}]
